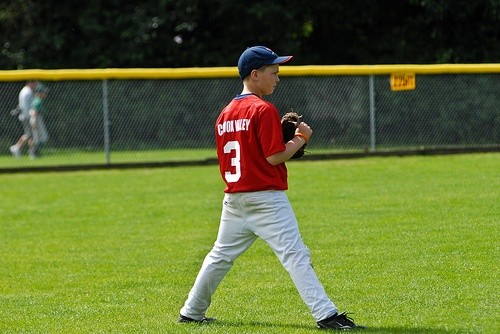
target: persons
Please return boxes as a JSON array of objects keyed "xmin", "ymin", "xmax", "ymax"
[
  {"xmin": 29, "ymin": 84, "xmax": 50, "ymax": 159},
  {"xmin": 177, "ymin": 45, "xmax": 365, "ymax": 332},
  {"xmin": 9, "ymin": 78, "xmax": 39, "ymax": 157}
]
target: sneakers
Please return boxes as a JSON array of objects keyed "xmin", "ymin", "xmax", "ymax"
[
  {"xmin": 317, "ymin": 311, "xmax": 366, "ymax": 330},
  {"xmin": 178, "ymin": 314, "xmax": 216, "ymax": 323}
]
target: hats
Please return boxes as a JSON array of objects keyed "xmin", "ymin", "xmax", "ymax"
[{"xmin": 238, "ymin": 46, "xmax": 294, "ymax": 81}]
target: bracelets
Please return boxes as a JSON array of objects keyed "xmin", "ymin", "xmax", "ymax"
[{"xmin": 294, "ymin": 131, "xmax": 308, "ymax": 141}]
